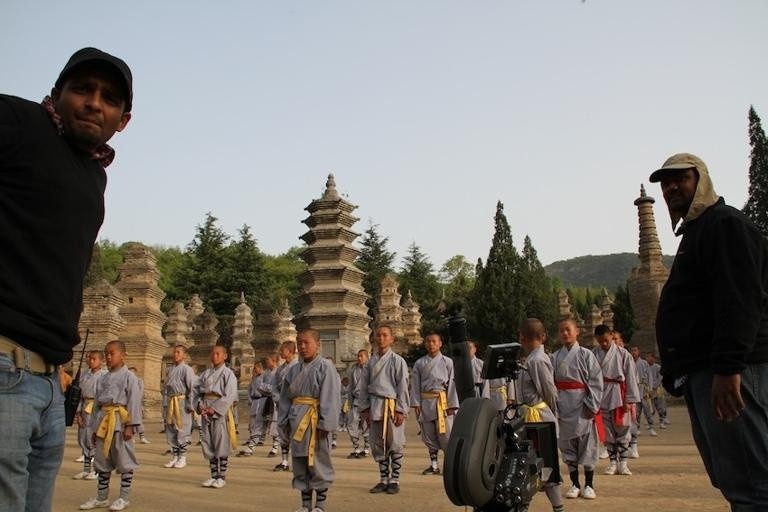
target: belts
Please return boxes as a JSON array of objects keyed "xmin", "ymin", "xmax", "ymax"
[{"xmin": 0, "ymin": 333, "xmax": 58, "ymax": 379}]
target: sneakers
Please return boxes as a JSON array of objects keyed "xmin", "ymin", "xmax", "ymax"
[
  {"xmin": 311, "ymin": 505, "xmax": 325, "ymax": 512},
  {"xmin": 632, "ymin": 417, "xmax": 673, "ymax": 438},
  {"xmin": 74, "ymin": 449, "xmax": 99, "ymax": 481},
  {"xmin": 294, "ymin": 503, "xmax": 311, "ymax": 512},
  {"xmin": 202, "ymin": 476, "xmax": 228, "ymax": 490},
  {"xmin": 330, "ymin": 440, "xmax": 374, "ymax": 462},
  {"xmin": 111, "ymin": 498, "xmax": 131, "ymax": 512},
  {"xmin": 79, "ymin": 494, "xmax": 110, "ymax": 512},
  {"xmin": 600, "ymin": 440, "xmax": 641, "ymax": 458},
  {"xmin": 137, "ymin": 429, "xmax": 294, "ymax": 473},
  {"xmin": 565, "ymin": 483, "xmax": 581, "ymax": 499},
  {"xmin": 582, "ymin": 485, "xmax": 598, "ymax": 499},
  {"xmin": 370, "ymin": 479, "xmax": 400, "ymax": 495},
  {"xmin": 422, "ymin": 465, "xmax": 440, "ymax": 475},
  {"xmin": 607, "ymin": 461, "xmax": 634, "ymax": 476}
]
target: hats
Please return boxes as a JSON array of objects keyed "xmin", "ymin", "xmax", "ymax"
[
  {"xmin": 54, "ymin": 44, "xmax": 135, "ymax": 114},
  {"xmin": 649, "ymin": 152, "xmax": 720, "ymax": 236}
]
